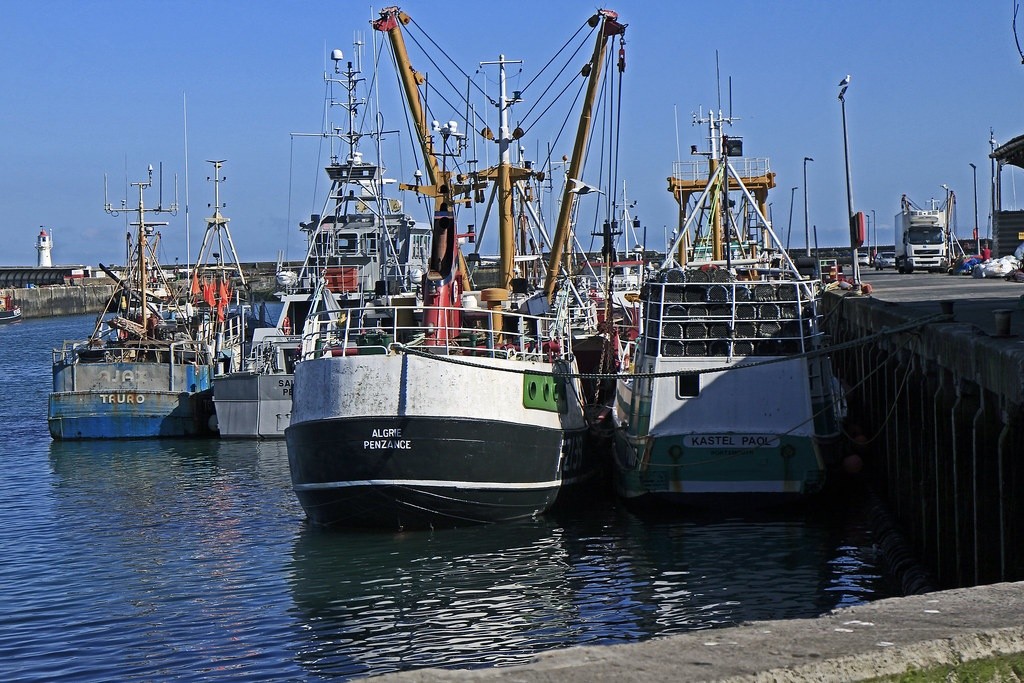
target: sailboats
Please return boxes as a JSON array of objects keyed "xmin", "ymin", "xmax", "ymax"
[{"xmin": 47, "ymin": 0, "xmax": 856, "ymax": 531}]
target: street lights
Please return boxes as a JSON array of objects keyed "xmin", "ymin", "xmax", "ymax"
[
  {"xmin": 941, "ymin": 184, "xmax": 950, "ymax": 235},
  {"xmin": 969, "ymin": 162, "xmax": 981, "ymax": 255},
  {"xmin": 866, "ymin": 214, "xmax": 870, "ymax": 258},
  {"xmin": 837, "ymin": 87, "xmax": 862, "ymax": 293},
  {"xmin": 871, "ymin": 209, "xmax": 878, "ymax": 255}
]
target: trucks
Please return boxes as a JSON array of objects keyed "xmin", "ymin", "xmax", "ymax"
[{"xmin": 894, "ymin": 210, "xmax": 948, "ymax": 275}]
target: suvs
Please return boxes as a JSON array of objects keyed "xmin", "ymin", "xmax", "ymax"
[{"xmin": 857, "ymin": 253, "xmax": 874, "ymax": 266}]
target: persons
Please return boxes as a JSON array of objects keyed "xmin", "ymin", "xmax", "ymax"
[{"xmin": 979, "ymin": 243, "xmax": 992, "ymax": 264}]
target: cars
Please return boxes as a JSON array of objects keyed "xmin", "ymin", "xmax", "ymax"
[{"xmin": 874, "ymin": 252, "xmax": 898, "ymax": 271}]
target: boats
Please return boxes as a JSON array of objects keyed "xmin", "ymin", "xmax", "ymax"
[{"xmin": 0, "ymin": 291, "xmax": 22, "ymax": 324}]
